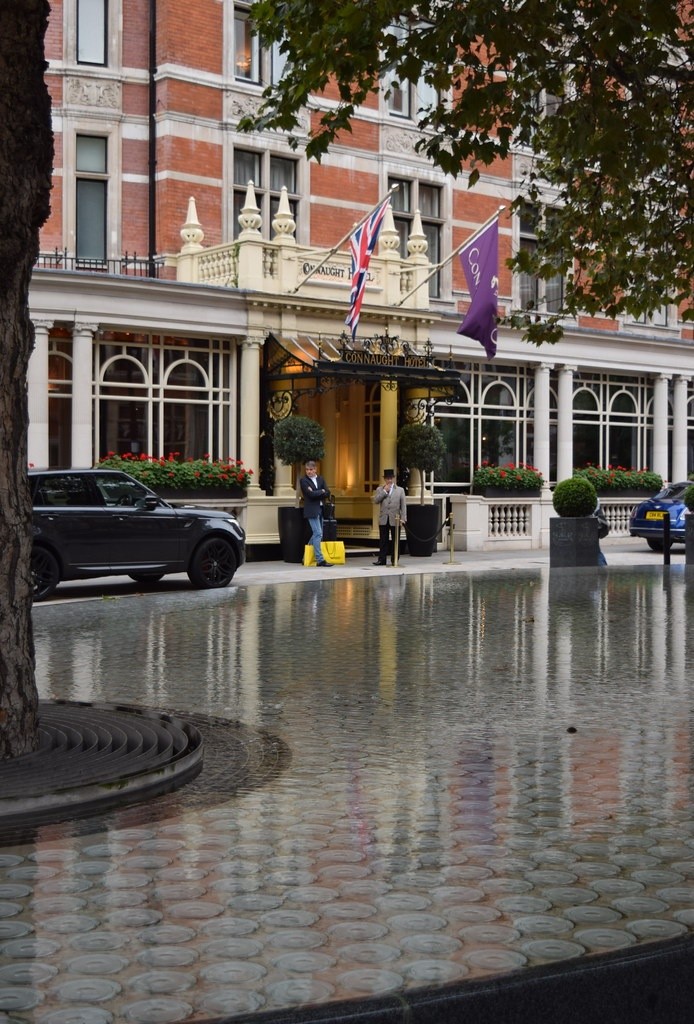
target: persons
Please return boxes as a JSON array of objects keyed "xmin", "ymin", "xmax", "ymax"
[
  {"xmin": 299, "ymin": 461, "xmax": 335, "ymax": 567},
  {"xmin": 372, "ymin": 469, "xmax": 407, "ymax": 566}
]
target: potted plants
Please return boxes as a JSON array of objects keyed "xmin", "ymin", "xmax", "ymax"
[
  {"xmin": 549, "ymin": 476, "xmax": 601, "ymax": 568},
  {"xmin": 272, "ymin": 415, "xmax": 325, "ymax": 563},
  {"xmin": 684, "ymin": 486, "xmax": 694, "ymax": 564},
  {"xmin": 397, "ymin": 424, "xmax": 447, "ymax": 556}
]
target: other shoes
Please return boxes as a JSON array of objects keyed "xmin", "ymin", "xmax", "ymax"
[
  {"xmin": 318, "ymin": 560, "xmax": 334, "ymax": 566},
  {"xmin": 372, "ymin": 561, "xmax": 386, "ymax": 566}
]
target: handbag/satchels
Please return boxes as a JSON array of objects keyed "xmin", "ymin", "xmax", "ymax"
[
  {"xmin": 320, "ymin": 541, "xmax": 345, "ymax": 565},
  {"xmin": 305, "ymin": 543, "xmax": 318, "ymax": 567}
]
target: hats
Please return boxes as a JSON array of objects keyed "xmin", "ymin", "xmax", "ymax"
[{"xmin": 382, "ymin": 469, "xmax": 396, "ymax": 477}]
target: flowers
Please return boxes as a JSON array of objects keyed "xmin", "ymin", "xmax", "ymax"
[
  {"xmin": 96, "ymin": 450, "xmax": 253, "ymax": 490},
  {"xmin": 473, "ymin": 459, "xmax": 546, "ymax": 492},
  {"xmin": 572, "ymin": 461, "xmax": 663, "ymax": 491}
]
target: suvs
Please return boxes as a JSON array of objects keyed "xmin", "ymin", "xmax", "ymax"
[{"xmin": 27, "ymin": 469, "xmax": 247, "ymax": 603}]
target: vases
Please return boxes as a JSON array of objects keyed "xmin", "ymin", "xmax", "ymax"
[
  {"xmin": 103, "ymin": 483, "xmax": 249, "ymax": 501},
  {"xmin": 596, "ymin": 488, "xmax": 660, "ymax": 498},
  {"xmin": 472, "ymin": 485, "xmax": 543, "ymax": 499}
]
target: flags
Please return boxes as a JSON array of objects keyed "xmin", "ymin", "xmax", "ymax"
[
  {"xmin": 457, "ymin": 214, "xmax": 499, "ymax": 361},
  {"xmin": 345, "ymin": 194, "xmax": 393, "ymax": 345}
]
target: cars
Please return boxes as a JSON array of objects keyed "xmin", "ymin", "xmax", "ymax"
[{"xmin": 629, "ymin": 480, "xmax": 694, "ymax": 553}]
